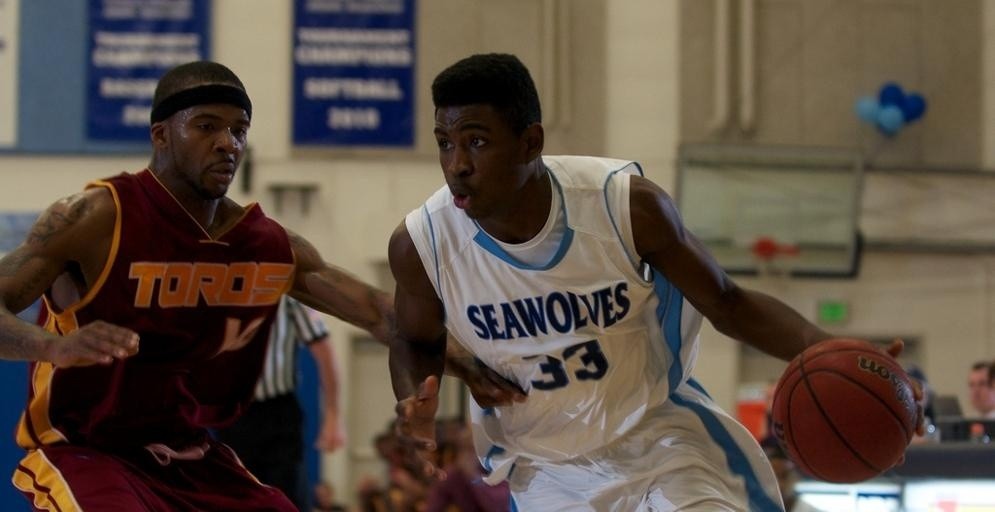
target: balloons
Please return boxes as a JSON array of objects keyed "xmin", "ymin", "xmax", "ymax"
[{"xmin": 852, "ymin": 77, "xmax": 926, "ymax": 136}]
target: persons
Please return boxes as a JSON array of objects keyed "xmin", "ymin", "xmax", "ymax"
[
  {"xmin": 385, "ymin": 52, "xmax": 928, "ymax": 510},
  {"xmin": 230, "ymin": 290, "xmax": 512, "ymax": 512},
  {"xmin": 0, "ymin": 61, "xmax": 529, "ymax": 511},
  {"xmin": 756, "ymin": 360, "xmax": 995, "ymax": 510}
]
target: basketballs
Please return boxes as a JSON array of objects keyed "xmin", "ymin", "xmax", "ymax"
[{"xmin": 770, "ymin": 339, "xmax": 916, "ymax": 483}]
